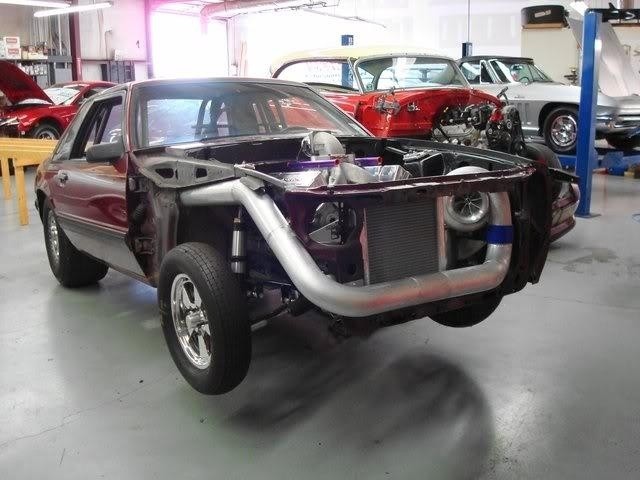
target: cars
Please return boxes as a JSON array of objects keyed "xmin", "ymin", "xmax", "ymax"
[{"xmin": 0, "ymin": 61, "xmax": 186, "ymax": 145}]
